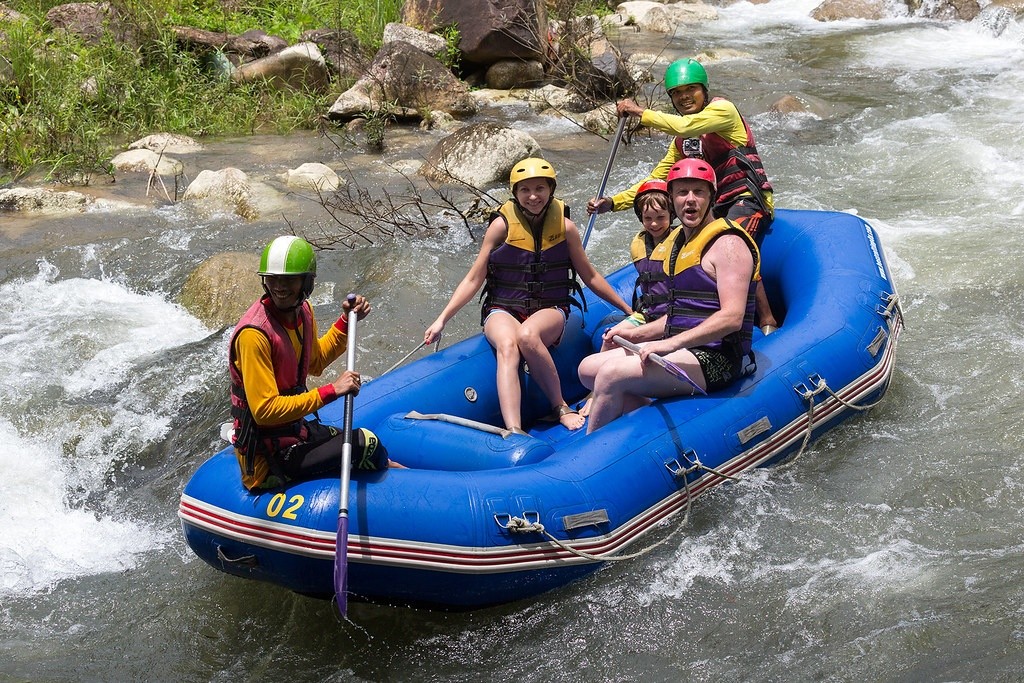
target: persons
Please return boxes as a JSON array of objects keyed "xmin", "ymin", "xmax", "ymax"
[
  {"xmin": 424, "ymin": 159, "xmax": 635, "ymax": 430},
  {"xmin": 578, "ymin": 180, "xmax": 676, "ymax": 417},
  {"xmin": 587, "ymin": 60, "xmax": 776, "ymax": 337},
  {"xmin": 228, "ymin": 236, "xmax": 409, "ymax": 492},
  {"xmin": 579, "ymin": 159, "xmax": 761, "ymax": 436}
]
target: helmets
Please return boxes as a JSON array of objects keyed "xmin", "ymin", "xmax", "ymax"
[
  {"xmin": 632, "ymin": 179, "xmax": 676, "ymax": 221},
  {"xmin": 666, "ymin": 58, "xmax": 710, "ymax": 95},
  {"xmin": 668, "ymin": 158, "xmax": 717, "ymax": 196},
  {"xmin": 510, "ymin": 157, "xmax": 556, "ymax": 193},
  {"xmin": 258, "ymin": 235, "xmax": 316, "ymax": 276}
]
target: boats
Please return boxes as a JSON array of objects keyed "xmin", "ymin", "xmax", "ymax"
[{"xmin": 175, "ymin": 210, "xmax": 903, "ymax": 613}]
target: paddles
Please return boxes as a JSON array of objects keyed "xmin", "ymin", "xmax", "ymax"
[
  {"xmin": 332, "ymin": 292, "xmax": 362, "ymax": 623},
  {"xmin": 568, "ymin": 110, "xmax": 631, "ymax": 295},
  {"xmin": 605, "ymin": 326, "xmax": 710, "ymax": 399}
]
